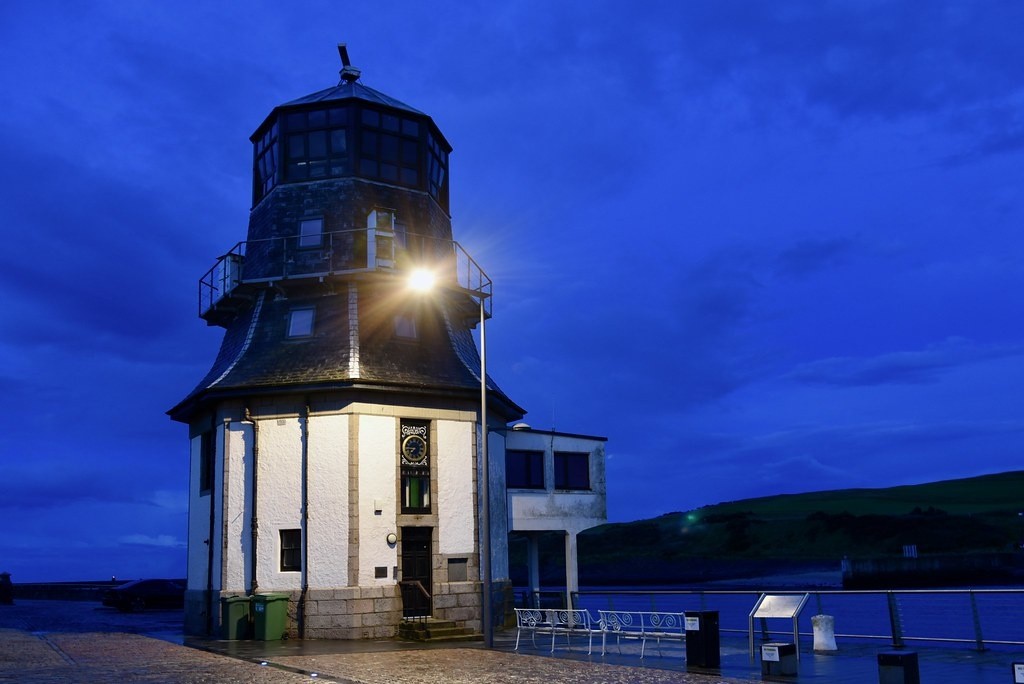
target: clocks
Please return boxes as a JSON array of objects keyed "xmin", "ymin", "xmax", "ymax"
[{"xmin": 402, "ymin": 435, "xmax": 426, "ymax": 462}]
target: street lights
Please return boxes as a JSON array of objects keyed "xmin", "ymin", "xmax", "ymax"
[{"xmin": 408, "ymin": 269, "xmax": 493, "ymax": 650}]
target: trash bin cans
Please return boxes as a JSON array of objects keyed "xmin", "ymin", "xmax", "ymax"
[
  {"xmin": 222, "ymin": 596, "xmax": 251, "ymax": 640},
  {"xmin": 683, "ymin": 610, "xmax": 721, "ymax": 676},
  {"xmin": 255, "ymin": 593, "xmax": 292, "ymax": 641}
]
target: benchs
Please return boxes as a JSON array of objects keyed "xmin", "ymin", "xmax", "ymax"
[
  {"xmin": 598, "ymin": 610, "xmax": 686, "ymax": 659},
  {"xmin": 514, "ymin": 608, "xmax": 607, "ymax": 655}
]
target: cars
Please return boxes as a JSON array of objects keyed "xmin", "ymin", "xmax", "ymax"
[{"xmin": 103, "ymin": 579, "xmax": 186, "ymax": 613}]
